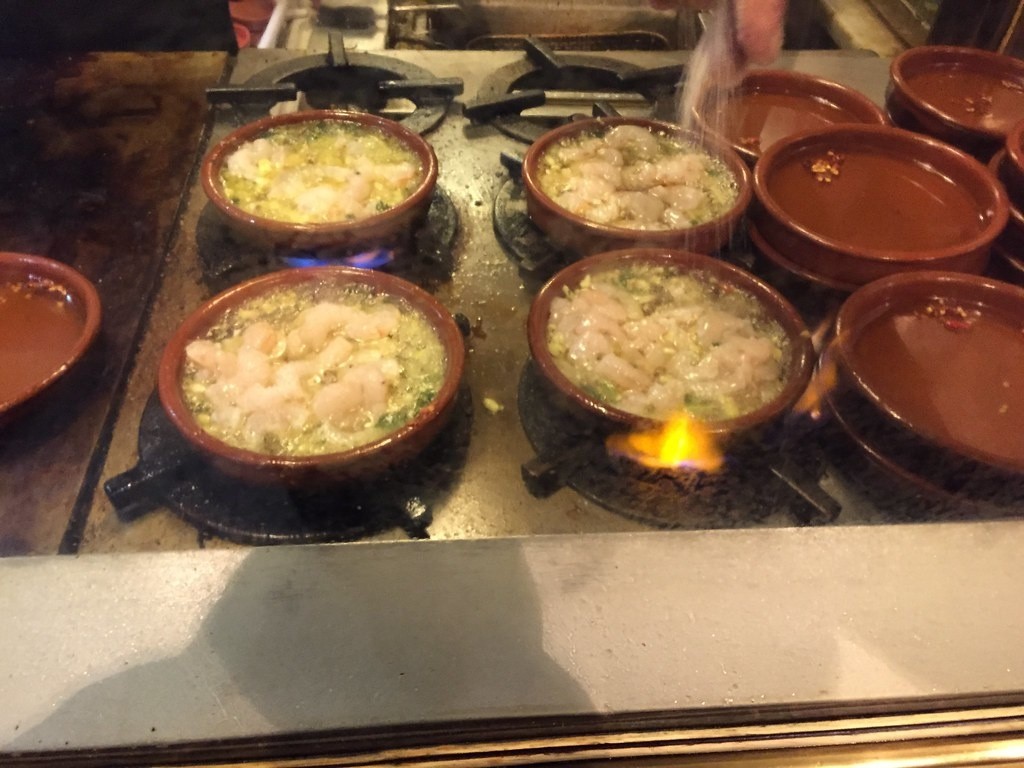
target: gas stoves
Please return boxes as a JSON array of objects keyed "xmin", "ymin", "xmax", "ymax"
[{"xmin": 74, "ymin": 48, "xmax": 843, "ymax": 559}]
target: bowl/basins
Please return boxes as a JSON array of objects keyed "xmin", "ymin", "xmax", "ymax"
[
  {"xmin": 157, "ymin": 264, "xmax": 465, "ymax": 492},
  {"xmin": 527, "ymin": 247, "xmax": 816, "ymax": 437},
  {"xmin": 0, "ymin": 250, "xmax": 102, "ymax": 428},
  {"xmin": 201, "ymin": 108, "xmax": 439, "ymax": 259},
  {"xmin": 522, "ymin": 116, "xmax": 755, "ymax": 256},
  {"xmin": 690, "ymin": 46, "xmax": 1024, "ymax": 519}
]
